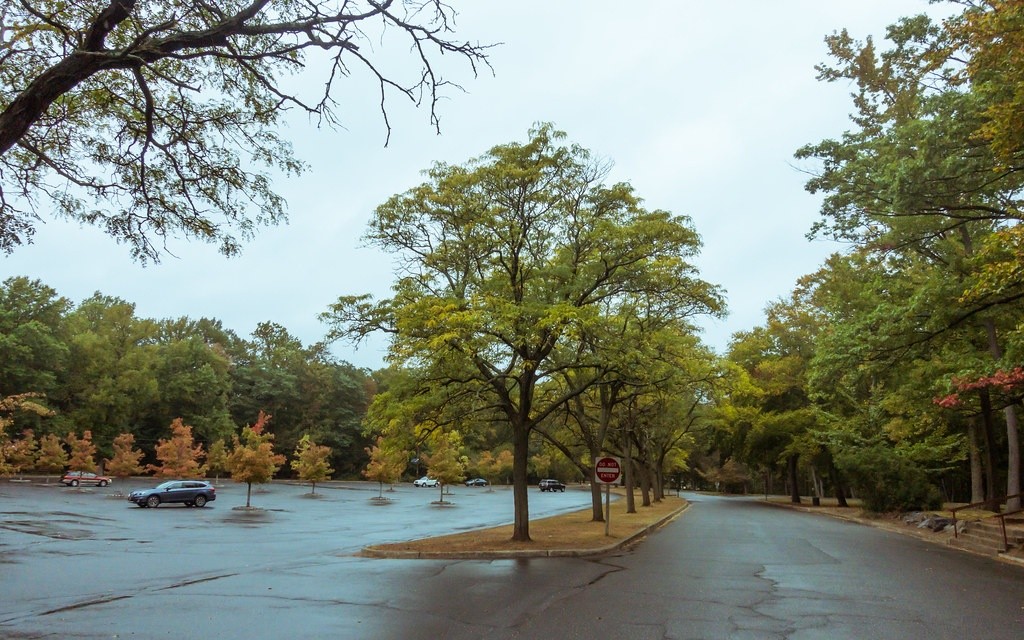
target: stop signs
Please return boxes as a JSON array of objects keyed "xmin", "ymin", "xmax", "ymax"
[{"xmin": 595, "ymin": 457, "xmax": 622, "ymax": 484}]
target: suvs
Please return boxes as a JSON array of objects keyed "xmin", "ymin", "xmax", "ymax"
[
  {"xmin": 413, "ymin": 476, "xmax": 439, "ymax": 487},
  {"xmin": 538, "ymin": 479, "xmax": 565, "ymax": 492},
  {"xmin": 128, "ymin": 480, "xmax": 216, "ymax": 508}
]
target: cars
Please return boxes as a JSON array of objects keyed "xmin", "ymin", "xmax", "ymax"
[
  {"xmin": 465, "ymin": 478, "xmax": 487, "ymax": 487},
  {"xmin": 59, "ymin": 472, "xmax": 112, "ymax": 487}
]
